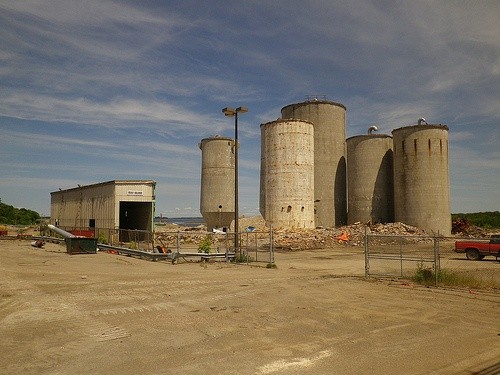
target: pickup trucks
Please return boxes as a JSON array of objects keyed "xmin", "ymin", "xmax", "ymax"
[{"xmin": 455, "ymin": 234, "xmax": 500, "ymax": 261}]
